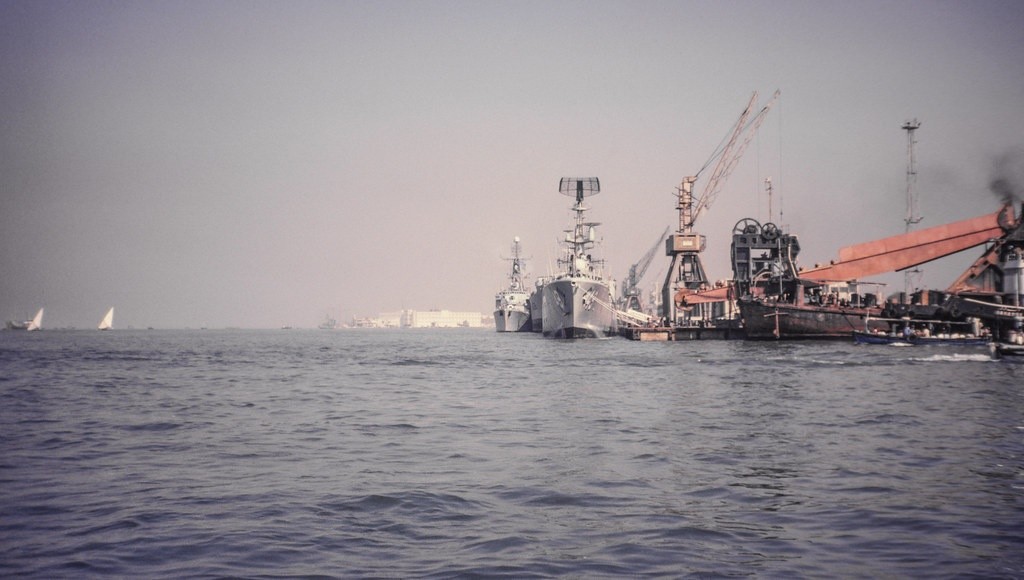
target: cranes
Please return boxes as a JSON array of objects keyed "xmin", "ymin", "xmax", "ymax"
[
  {"xmin": 663, "ymin": 89, "xmax": 782, "ymax": 326},
  {"xmin": 621, "ymin": 224, "xmax": 670, "ymax": 324}
]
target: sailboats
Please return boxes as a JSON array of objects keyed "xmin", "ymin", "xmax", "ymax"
[
  {"xmin": 98, "ymin": 307, "xmax": 114, "ymax": 330},
  {"xmin": 9, "ymin": 308, "xmax": 44, "ymax": 330}
]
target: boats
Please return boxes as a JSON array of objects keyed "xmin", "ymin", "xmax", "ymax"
[
  {"xmin": 738, "ymin": 285, "xmax": 889, "ymax": 340},
  {"xmin": 850, "ymin": 329, "xmax": 992, "ymax": 345},
  {"xmin": 532, "ymin": 177, "xmax": 618, "ymax": 339},
  {"xmin": 493, "ymin": 236, "xmax": 531, "ymax": 331}
]
